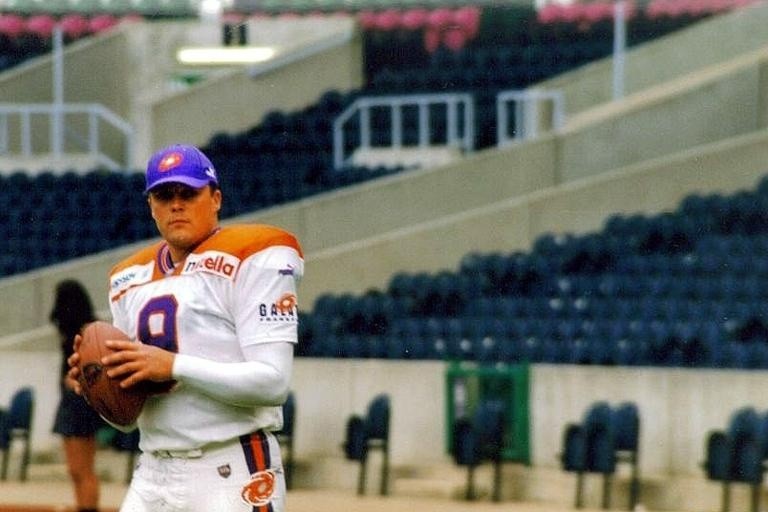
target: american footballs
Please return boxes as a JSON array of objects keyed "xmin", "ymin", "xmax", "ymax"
[{"xmin": 74, "ymin": 320, "xmax": 147, "ymax": 425}]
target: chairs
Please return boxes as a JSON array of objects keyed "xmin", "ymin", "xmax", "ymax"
[
  {"xmin": 286, "ymin": 180, "xmax": 767, "ymax": 512},
  {"xmin": 1, "ymin": 389, "xmax": 34, "ymax": 481}
]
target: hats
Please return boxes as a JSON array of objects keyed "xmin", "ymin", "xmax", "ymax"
[{"xmin": 142, "ymin": 143, "xmax": 219, "ymax": 196}]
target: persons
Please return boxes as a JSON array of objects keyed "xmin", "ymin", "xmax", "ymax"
[
  {"xmin": 66, "ymin": 147, "xmax": 304, "ymax": 512},
  {"xmin": 48, "ymin": 280, "xmax": 100, "ymax": 511}
]
